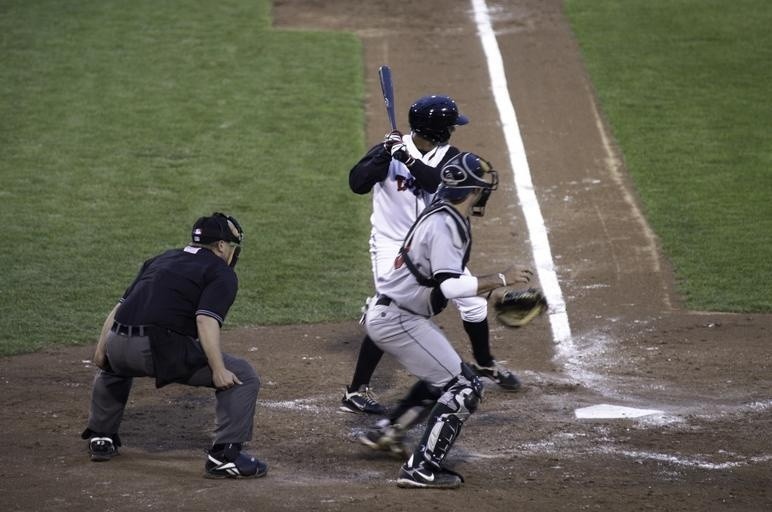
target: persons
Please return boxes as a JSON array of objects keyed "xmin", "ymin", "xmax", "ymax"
[
  {"xmin": 359, "ymin": 152, "xmax": 535, "ymax": 488},
  {"xmin": 81, "ymin": 212, "xmax": 269, "ymax": 479},
  {"xmin": 341, "ymin": 94, "xmax": 520, "ymax": 414}
]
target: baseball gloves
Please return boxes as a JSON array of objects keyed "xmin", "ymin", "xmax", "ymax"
[{"xmin": 493, "ymin": 288, "xmax": 547, "ymax": 329}]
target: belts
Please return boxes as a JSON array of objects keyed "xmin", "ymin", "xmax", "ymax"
[{"xmin": 111, "ymin": 321, "xmax": 152, "ymax": 337}]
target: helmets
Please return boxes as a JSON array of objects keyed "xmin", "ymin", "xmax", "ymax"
[
  {"xmin": 408, "ymin": 95, "xmax": 470, "ymax": 137},
  {"xmin": 440, "ymin": 151, "xmax": 499, "ymax": 205},
  {"xmin": 191, "ymin": 212, "xmax": 244, "ymax": 244}
]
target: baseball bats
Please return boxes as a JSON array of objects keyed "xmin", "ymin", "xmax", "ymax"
[{"xmin": 378, "ymin": 65, "xmax": 397, "ymax": 130}]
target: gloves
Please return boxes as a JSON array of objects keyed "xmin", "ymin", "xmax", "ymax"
[{"xmin": 384, "ymin": 129, "xmax": 410, "ymax": 163}]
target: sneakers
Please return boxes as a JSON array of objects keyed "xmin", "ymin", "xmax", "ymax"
[
  {"xmin": 339, "ymin": 385, "xmax": 388, "ymax": 415},
  {"xmin": 359, "ymin": 424, "xmax": 465, "ymax": 489},
  {"xmin": 88, "ymin": 432, "xmax": 117, "ymax": 462},
  {"xmin": 204, "ymin": 442, "xmax": 270, "ymax": 479},
  {"xmin": 470, "ymin": 360, "xmax": 522, "ymax": 393}
]
